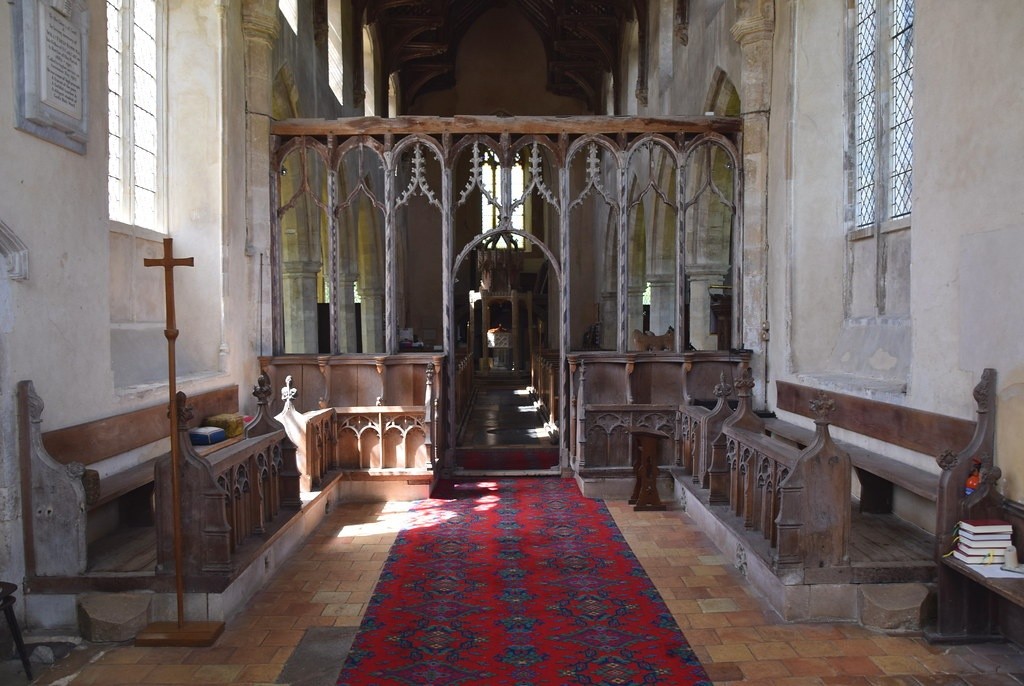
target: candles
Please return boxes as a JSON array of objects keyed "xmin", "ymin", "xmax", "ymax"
[{"xmin": 1004, "ymin": 546, "xmax": 1018, "ymax": 566}]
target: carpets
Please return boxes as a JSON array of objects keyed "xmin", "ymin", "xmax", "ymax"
[
  {"xmin": 336, "ymin": 474, "xmax": 711, "ymax": 686},
  {"xmin": 453, "ymin": 448, "xmax": 559, "ymax": 470}
]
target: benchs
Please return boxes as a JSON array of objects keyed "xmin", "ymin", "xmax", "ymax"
[
  {"xmin": 760, "ymin": 379, "xmax": 976, "ymax": 497},
  {"xmin": 925, "ymin": 465, "xmax": 1024, "ymax": 644},
  {"xmin": 42, "ymin": 386, "xmax": 241, "ymax": 510}
]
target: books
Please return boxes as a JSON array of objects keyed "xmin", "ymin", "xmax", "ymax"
[{"xmin": 953, "ymin": 519, "xmax": 1013, "ymax": 564}]
